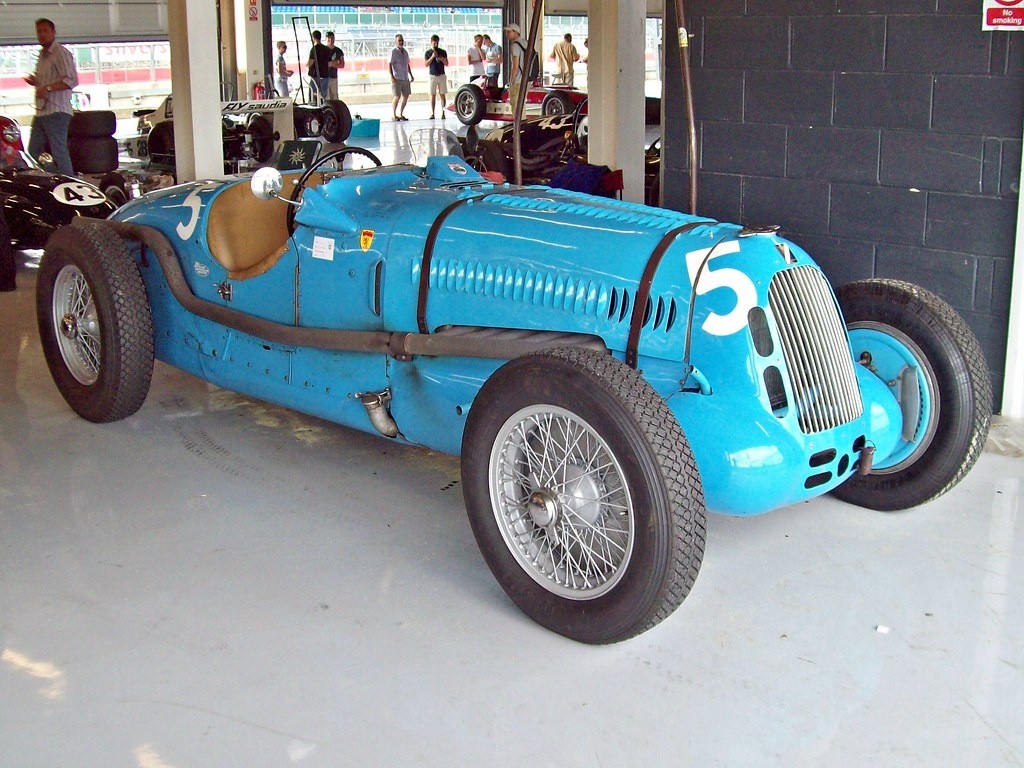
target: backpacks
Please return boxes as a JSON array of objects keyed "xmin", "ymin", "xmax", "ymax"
[{"xmin": 513, "ymin": 40, "xmax": 539, "ymax": 82}]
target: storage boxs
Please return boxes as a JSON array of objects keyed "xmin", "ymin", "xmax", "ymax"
[{"xmin": 350, "ymin": 118, "xmax": 381, "ymax": 137}]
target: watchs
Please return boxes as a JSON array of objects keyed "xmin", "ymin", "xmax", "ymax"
[{"xmin": 46, "ymin": 84, "xmax": 53, "ymax": 94}]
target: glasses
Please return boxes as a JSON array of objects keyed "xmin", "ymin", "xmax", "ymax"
[
  {"xmin": 28, "ymin": 95, "xmax": 48, "ymax": 112},
  {"xmin": 396, "ymin": 40, "xmax": 403, "ymax": 42},
  {"xmin": 280, "ymin": 46, "xmax": 286, "ymax": 49}
]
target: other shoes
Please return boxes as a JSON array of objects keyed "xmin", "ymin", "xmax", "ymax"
[
  {"xmin": 430, "ymin": 115, "xmax": 435, "ymax": 119},
  {"xmin": 394, "ymin": 116, "xmax": 408, "ymax": 121},
  {"xmin": 441, "ymin": 116, "xmax": 445, "ymax": 119}
]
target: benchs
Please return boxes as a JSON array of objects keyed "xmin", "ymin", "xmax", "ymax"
[{"xmin": 207, "ymin": 170, "xmax": 324, "ymax": 278}]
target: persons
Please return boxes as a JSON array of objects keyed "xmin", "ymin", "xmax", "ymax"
[
  {"xmin": 389, "ymin": 34, "xmax": 415, "ymax": 121},
  {"xmin": 549, "ymin": 34, "xmax": 588, "ymax": 86},
  {"xmin": 504, "ymin": 24, "xmax": 532, "ymax": 121},
  {"xmin": 273, "ymin": 41, "xmax": 294, "ymax": 97},
  {"xmin": 468, "ymin": 34, "xmax": 502, "ymax": 88},
  {"xmin": 24, "ymin": 18, "xmax": 79, "ymax": 177},
  {"xmin": 425, "ymin": 35, "xmax": 448, "ymax": 119},
  {"xmin": 306, "ymin": 31, "xmax": 345, "ymax": 102}
]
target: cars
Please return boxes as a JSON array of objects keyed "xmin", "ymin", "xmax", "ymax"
[
  {"xmin": 123, "ymin": 86, "xmax": 352, "ymax": 166},
  {"xmin": 36, "ymin": 132, "xmax": 995, "ymax": 647},
  {"xmin": 0, "ymin": 115, "xmax": 130, "ymax": 286},
  {"xmin": 468, "ymin": 99, "xmax": 663, "ymax": 205},
  {"xmin": 443, "ymin": 70, "xmax": 591, "ymax": 125}
]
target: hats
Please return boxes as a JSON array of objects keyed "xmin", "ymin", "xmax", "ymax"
[{"xmin": 504, "ymin": 23, "xmax": 521, "ymax": 37}]
